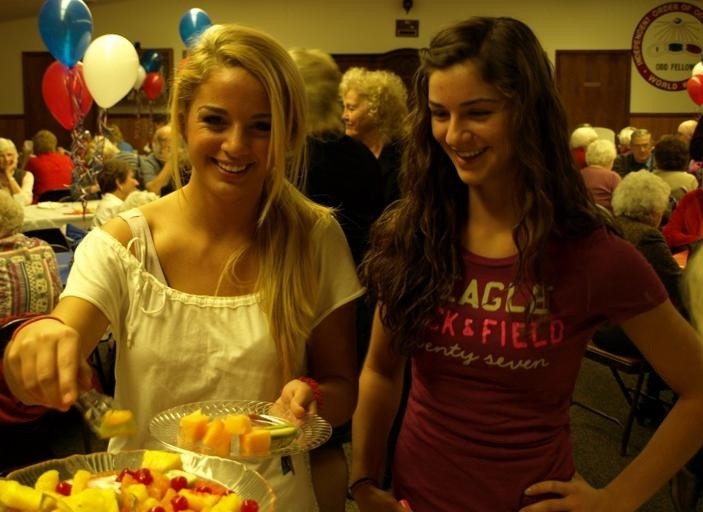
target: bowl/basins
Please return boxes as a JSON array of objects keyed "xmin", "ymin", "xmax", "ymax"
[{"xmin": 2, "ymin": 448, "xmax": 279, "ymax": 512}]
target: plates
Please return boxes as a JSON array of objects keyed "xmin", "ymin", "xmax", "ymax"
[
  {"xmin": 150, "ymin": 398, "xmax": 331, "ymax": 461},
  {"xmin": 35, "ymin": 200, "xmax": 61, "ymax": 210}
]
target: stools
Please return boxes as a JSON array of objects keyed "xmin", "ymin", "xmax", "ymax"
[{"xmin": 571, "ymin": 337, "xmax": 675, "ymax": 459}]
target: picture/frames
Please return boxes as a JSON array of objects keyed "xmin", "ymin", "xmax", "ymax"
[{"xmin": 107, "ymin": 47, "xmax": 176, "ymax": 118}]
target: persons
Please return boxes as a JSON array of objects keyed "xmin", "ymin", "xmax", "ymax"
[
  {"xmin": 0, "ymin": 124, "xmax": 192, "ymax": 321},
  {"xmin": 0, "ymin": 24, "xmax": 367, "ymax": 512},
  {"xmin": 570, "ymin": 116, "xmax": 702, "ymax": 430},
  {"xmin": 346, "ymin": 17, "xmax": 703, "ymax": 512},
  {"xmin": 282, "ymin": 47, "xmax": 410, "ymax": 511}
]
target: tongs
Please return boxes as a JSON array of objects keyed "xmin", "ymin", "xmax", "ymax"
[{"xmin": 69, "ymin": 377, "xmax": 132, "ymax": 440}]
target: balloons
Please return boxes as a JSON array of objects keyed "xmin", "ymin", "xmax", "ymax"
[
  {"xmin": 179, "ymin": 8, "xmax": 213, "ymax": 48},
  {"xmin": 687, "ymin": 74, "xmax": 703, "ymax": 106},
  {"xmin": 686, "ymin": 61, "xmax": 703, "ymax": 75},
  {"xmin": 42, "ymin": 0, "xmax": 163, "ymax": 130}
]
